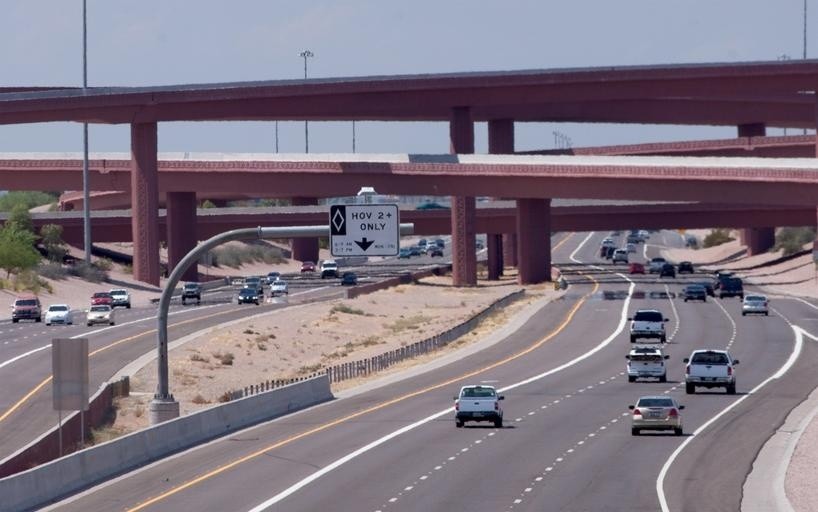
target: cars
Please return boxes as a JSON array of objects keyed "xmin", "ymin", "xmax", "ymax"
[
  {"xmin": 297, "ymin": 260, "xmax": 358, "ymax": 288},
  {"xmin": 414, "ymin": 203, "xmax": 447, "ymax": 208},
  {"xmin": 225, "ymin": 271, "xmax": 289, "ymax": 306},
  {"xmin": 626, "ymin": 309, "xmax": 739, "ymax": 436},
  {"xmin": 600, "ymin": 230, "xmax": 675, "ymax": 278},
  {"xmin": 684, "ymin": 278, "xmax": 744, "ymax": 302},
  {"xmin": 180, "ymin": 283, "xmax": 203, "ymax": 301},
  {"xmin": 474, "ymin": 236, "xmax": 486, "ymax": 250},
  {"xmin": 453, "ymin": 384, "xmax": 505, "ymax": 428},
  {"xmin": 10, "ymin": 289, "xmax": 131, "ymax": 328},
  {"xmin": 398, "ymin": 238, "xmax": 445, "ymax": 258},
  {"xmin": 741, "ymin": 295, "xmax": 770, "ymax": 316},
  {"xmin": 678, "ymin": 261, "xmax": 694, "ymax": 274}
]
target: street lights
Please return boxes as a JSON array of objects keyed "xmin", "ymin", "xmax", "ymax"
[{"xmin": 297, "ymin": 50, "xmax": 314, "ymax": 153}]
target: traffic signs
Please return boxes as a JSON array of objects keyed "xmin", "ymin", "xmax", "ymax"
[{"xmin": 330, "ymin": 204, "xmax": 399, "ymax": 256}]
